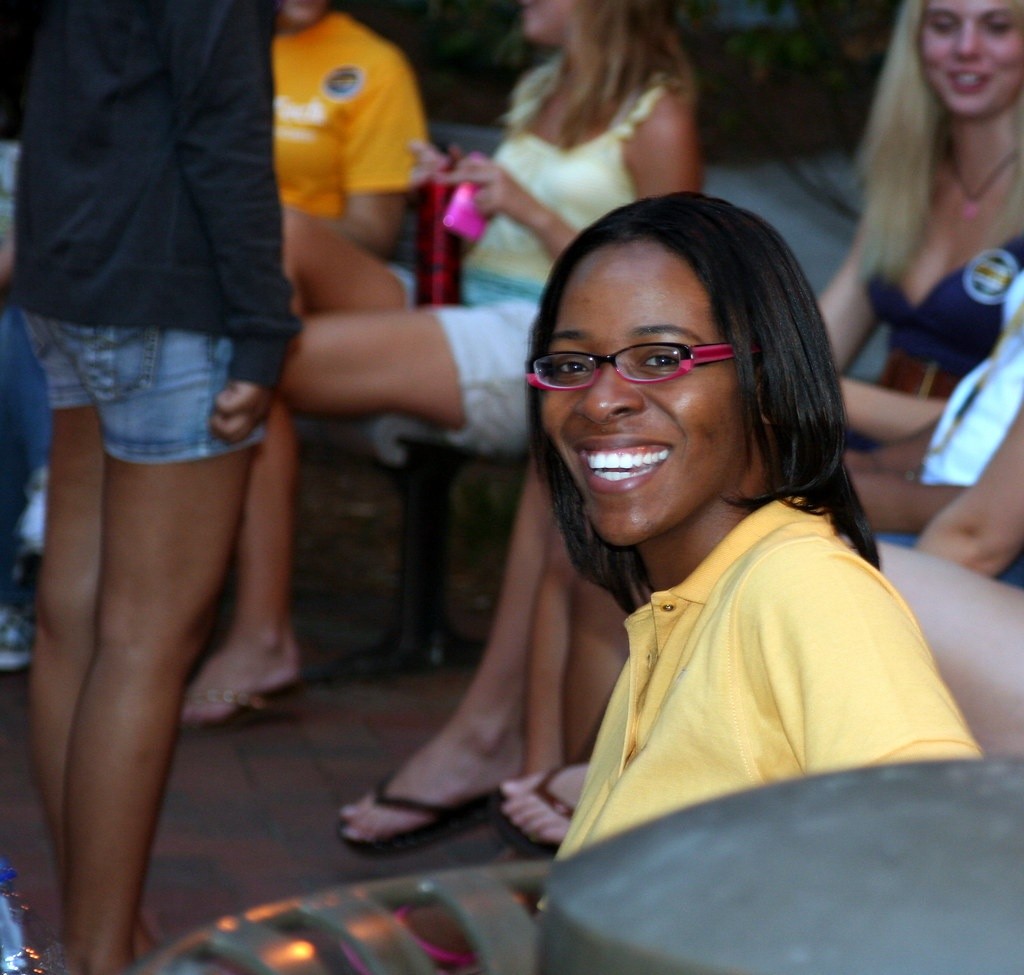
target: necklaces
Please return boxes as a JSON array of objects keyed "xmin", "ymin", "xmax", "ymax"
[{"xmin": 953, "ymin": 152, "xmax": 1017, "ymax": 223}]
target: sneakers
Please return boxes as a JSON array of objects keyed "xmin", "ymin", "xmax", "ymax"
[{"xmin": 0, "ymin": 607, "xmax": 33, "ymax": 671}]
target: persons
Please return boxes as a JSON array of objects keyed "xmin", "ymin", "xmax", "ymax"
[
  {"xmin": 0, "ymin": 0, "xmax": 1024, "ymax": 974},
  {"xmin": 526, "ymin": 191, "xmax": 987, "ymax": 856}
]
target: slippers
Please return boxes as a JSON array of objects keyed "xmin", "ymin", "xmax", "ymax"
[
  {"xmin": 338, "ymin": 774, "xmax": 499, "ymax": 855},
  {"xmin": 491, "ymin": 764, "xmax": 574, "ymax": 855},
  {"xmin": 173, "ymin": 684, "xmax": 300, "ymax": 737}
]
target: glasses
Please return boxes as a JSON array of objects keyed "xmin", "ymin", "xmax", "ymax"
[{"xmin": 526, "ymin": 343, "xmax": 760, "ymax": 390}]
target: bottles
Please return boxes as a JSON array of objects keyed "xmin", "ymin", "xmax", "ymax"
[{"xmin": 0, "ymin": 855, "xmax": 70, "ymax": 975}]
